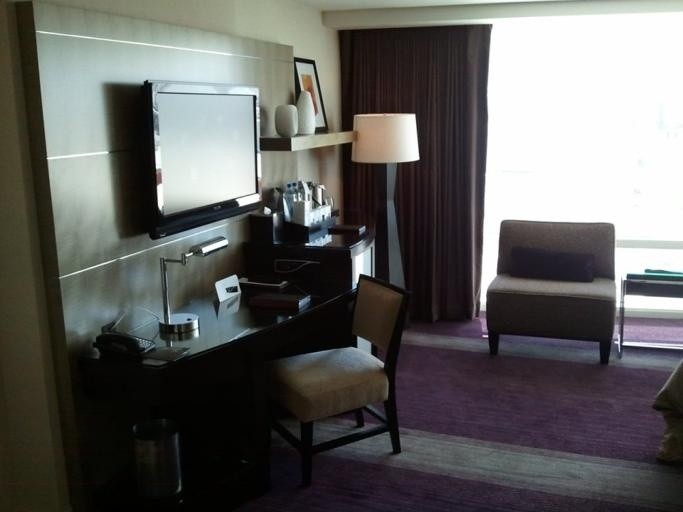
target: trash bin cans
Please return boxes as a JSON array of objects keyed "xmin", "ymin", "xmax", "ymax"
[{"xmin": 133, "ymin": 420, "xmax": 183, "ymax": 499}]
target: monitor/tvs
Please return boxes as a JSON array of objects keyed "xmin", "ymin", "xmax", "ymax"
[{"xmin": 141, "ymin": 78, "xmax": 264, "ymax": 241}]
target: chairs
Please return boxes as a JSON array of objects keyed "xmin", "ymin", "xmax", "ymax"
[
  {"xmin": 251, "ymin": 274, "xmax": 415, "ymax": 494},
  {"xmin": 487, "ymin": 218, "xmax": 616, "ymax": 365}
]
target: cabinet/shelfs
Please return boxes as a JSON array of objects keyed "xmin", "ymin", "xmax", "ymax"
[{"xmin": 243, "ymin": 206, "xmax": 378, "ymax": 360}]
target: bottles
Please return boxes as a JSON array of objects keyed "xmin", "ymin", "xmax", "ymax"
[{"xmin": 286, "ymin": 183, "xmax": 298, "ymax": 217}]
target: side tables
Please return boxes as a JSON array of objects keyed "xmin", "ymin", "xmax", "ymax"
[{"xmin": 617, "ymin": 278, "xmax": 683, "ymax": 359}]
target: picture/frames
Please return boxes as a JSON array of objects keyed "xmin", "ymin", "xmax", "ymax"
[{"xmin": 292, "ymin": 55, "xmax": 329, "ymax": 135}]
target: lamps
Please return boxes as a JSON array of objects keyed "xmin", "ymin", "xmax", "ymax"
[
  {"xmin": 350, "ymin": 112, "xmax": 420, "ymax": 332},
  {"xmin": 158, "ymin": 235, "xmax": 230, "ymax": 336}
]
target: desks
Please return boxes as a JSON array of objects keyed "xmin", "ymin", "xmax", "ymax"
[{"xmin": 91, "ymin": 273, "xmax": 360, "ymax": 512}]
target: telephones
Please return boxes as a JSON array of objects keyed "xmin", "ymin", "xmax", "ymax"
[{"xmin": 93, "ymin": 333, "xmax": 158, "ymax": 357}]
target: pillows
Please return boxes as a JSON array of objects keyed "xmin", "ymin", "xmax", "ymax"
[{"xmin": 508, "ymin": 246, "xmax": 594, "ymax": 283}]
